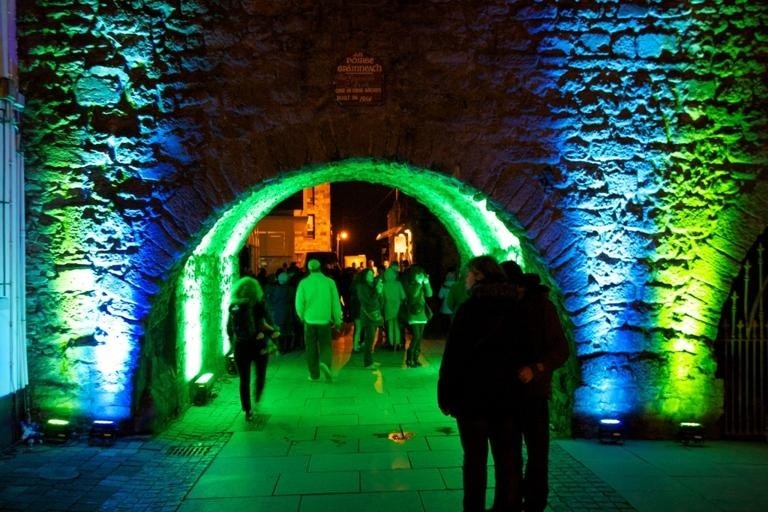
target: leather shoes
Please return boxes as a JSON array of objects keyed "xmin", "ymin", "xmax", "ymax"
[{"xmin": 405, "ymin": 358, "xmax": 421, "ymax": 367}]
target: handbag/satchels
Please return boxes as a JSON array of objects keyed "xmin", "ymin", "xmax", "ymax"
[
  {"xmin": 398, "ymin": 304, "xmax": 407, "ymax": 323},
  {"xmin": 425, "ymin": 304, "xmax": 433, "ymax": 320},
  {"xmin": 367, "ymin": 310, "xmax": 383, "ymax": 321}
]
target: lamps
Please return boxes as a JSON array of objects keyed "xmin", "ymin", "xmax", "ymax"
[
  {"xmin": 226, "ymin": 353, "xmax": 238, "ymax": 375},
  {"xmin": 193, "ymin": 372, "xmax": 217, "ymax": 406},
  {"xmin": 679, "ymin": 422, "xmax": 705, "ymax": 448},
  {"xmin": 597, "ymin": 417, "xmax": 624, "ymax": 446},
  {"xmin": 88, "ymin": 420, "xmax": 116, "ymax": 447},
  {"xmin": 44, "ymin": 419, "xmax": 71, "ymax": 447}
]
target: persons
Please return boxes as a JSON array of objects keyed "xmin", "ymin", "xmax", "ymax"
[
  {"xmin": 437, "ymin": 270, "xmax": 455, "ymax": 341},
  {"xmin": 500, "ymin": 259, "xmax": 572, "ymax": 510},
  {"xmin": 226, "ymin": 274, "xmax": 283, "ymax": 423},
  {"xmin": 253, "ymin": 257, "xmax": 433, "ymax": 383},
  {"xmin": 435, "ymin": 254, "xmax": 524, "ymax": 511}
]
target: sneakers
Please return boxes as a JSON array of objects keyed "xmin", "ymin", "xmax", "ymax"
[
  {"xmin": 308, "ymin": 376, "xmax": 320, "ymax": 382},
  {"xmin": 367, "ymin": 362, "xmax": 381, "ymax": 369},
  {"xmin": 320, "ymin": 362, "xmax": 332, "ymax": 378}
]
